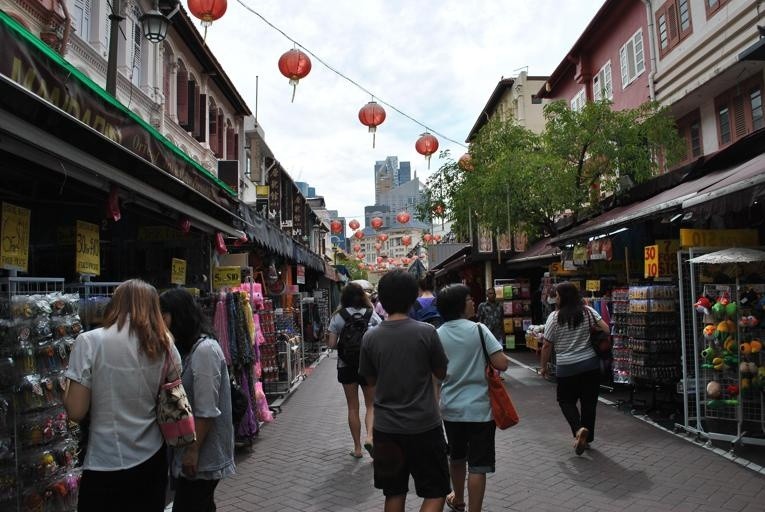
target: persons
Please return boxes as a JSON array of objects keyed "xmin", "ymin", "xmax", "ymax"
[
  {"xmin": 60, "ymin": 277, "xmax": 184, "ymax": 512},
  {"xmin": 409, "ymin": 277, "xmax": 443, "ymax": 329},
  {"xmin": 158, "ymin": 286, "xmax": 237, "ymax": 512},
  {"xmin": 328, "ymin": 282, "xmax": 388, "ymax": 458},
  {"xmin": 538, "ymin": 282, "xmax": 611, "ymax": 455},
  {"xmin": 434, "ymin": 283, "xmax": 508, "ymax": 512},
  {"xmin": 359, "ymin": 271, "xmax": 453, "ymax": 512}
]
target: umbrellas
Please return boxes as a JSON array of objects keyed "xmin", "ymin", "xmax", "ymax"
[{"xmin": 684, "ymin": 239, "xmax": 765, "ymax": 278}]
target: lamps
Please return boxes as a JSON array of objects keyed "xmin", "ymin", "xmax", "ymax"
[{"xmin": 138, "ymin": 0, "xmax": 169, "ymax": 43}]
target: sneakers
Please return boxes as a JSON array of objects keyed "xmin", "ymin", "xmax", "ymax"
[{"xmin": 573, "ymin": 426, "xmax": 591, "ymax": 456}]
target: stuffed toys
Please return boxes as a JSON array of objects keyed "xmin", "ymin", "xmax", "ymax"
[{"xmin": 693, "ymin": 285, "xmax": 765, "ymax": 410}]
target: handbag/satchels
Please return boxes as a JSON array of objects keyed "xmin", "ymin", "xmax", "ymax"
[
  {"xmin": 154, "ymin": 380, "xmax": 198, "ymax": 451},
  {"xmin": 304, "ymin": 319, "xmax": 327, "ymax": 343},
  {"xmin": 483, "ymin": 362, "xmax": 519, "ymax": 430},
  {"xmin": 590, "ymin": 325, "xmax": 615, "ymax": 359},
  {"xmin": 561, "ymin": 238, "xmax": 612, "ymax": 272},
  {"xmin": 227, "ymin": 370, "xmax": 248, "ymax": 426}
]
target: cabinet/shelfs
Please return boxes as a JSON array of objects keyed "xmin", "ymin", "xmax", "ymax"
[
  {"xmin": 540, "ymin": 244, "xmax": 695, "ymax": 414},
  {"xmin": 0, "ymin": 268, "xmax": 330, "ymax": 512}
]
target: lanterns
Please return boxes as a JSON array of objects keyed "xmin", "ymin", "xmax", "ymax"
[
  {"xmin": 459, "ymin": 152, "xmax": 473, "ymax": 172},
  {"xmin": 331, "ymin": 221, "xmax": 343, "ymax": 234},
  {"xmin": 186, "ymin": 0, "xmax": 227, "ymax": 48},
  {"xmin": 371, "ymin": 217, "xmax": 383, "ymax": 232},
  {"xmin": 397, "ymin": 211, "xmax": 410, "ymax": 224},
  {"xmin": 348, "ymin": 220, "xmax": 361, "ymax": 232},
  {"xmin": 358, "ymin": 101, "xmax": 386, "ymax": 149},
  {"xmin": 415, "ymin": 133, "xmax": 439, "ymax": 169},
  {"xmin": 435, "ymin": 206, "xmax": 442, "ymax": 214},
  {"xmin": 277, "ymin": 47, "xmax": 312, "ymax": 104},
  {"xmin": 352, "ymin": 230, "xmax": 441, "ymax": 271}
]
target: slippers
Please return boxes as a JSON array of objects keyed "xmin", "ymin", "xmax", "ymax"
[
  {"xmin": 349, "ymin": 448, "xmax": 363, "ymax": 458},
  {"xmin": 364, "ymin": 444, "xmax": 372, "ymax": 455}
]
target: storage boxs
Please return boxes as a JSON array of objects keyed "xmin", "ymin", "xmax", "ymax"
[{"xmin": 526, "ymin": 335, "xmax": 541, "ymax": 350}]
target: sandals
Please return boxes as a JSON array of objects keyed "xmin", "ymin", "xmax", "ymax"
[{"xmin": 446, "ymin": 493, "xmax": 466, "ymax": 512}]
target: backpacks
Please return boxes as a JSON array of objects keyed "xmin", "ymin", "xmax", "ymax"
[
  {"xmin": 412, "ymin": 296, "xmax": 444, "ymax": 330},
  {"xmin": 337, "ymin": 306, "xmax": 373, "ymax": 367}
]
